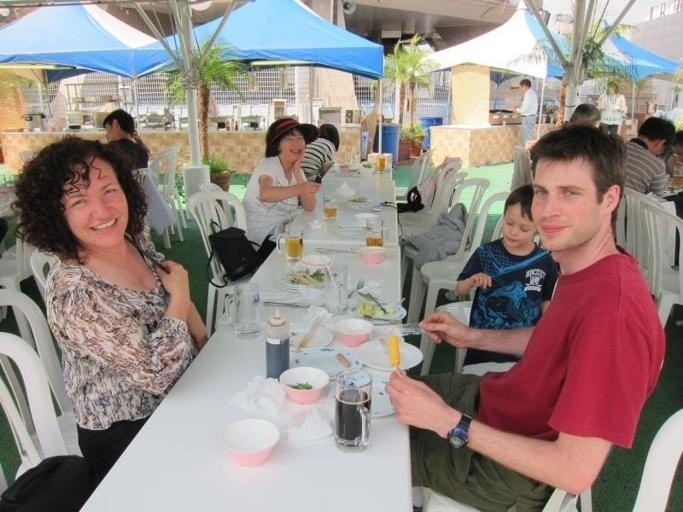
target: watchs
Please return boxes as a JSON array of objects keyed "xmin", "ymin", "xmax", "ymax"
[{"xmin": 445, "ymin": 412, "xmax": 472, "ymax": 450}]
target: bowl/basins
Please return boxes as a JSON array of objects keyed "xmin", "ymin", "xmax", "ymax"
[
  {"xmin": 216, "ymin": 419, "xmax": 284, "ymax": 468},
  {"xmin": 358, "ymin": 245, "xmax": 385, "ymax": 266},
  {"xmin": 337, "ymin": 165, "xmax": 351, "ymax": 173},
  {"xmin": 276, "ymin": 366, "xmax": 330, "ymax": 406},
  {"xmin": 333, "ymin": 317, "xmax": 376, "ymax": 348}
]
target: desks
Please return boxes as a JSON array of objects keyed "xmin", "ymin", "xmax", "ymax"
[
  {"xmin": 80, "ymin": 163, "xmax": 416, "ymax": 509},
  {"xmin": 1, "ymin": 124, "xmax": 361, "ymax": 173},
  {"xmin": 425, "ymin": 119, "xmax": 553, "ymax": 168}
]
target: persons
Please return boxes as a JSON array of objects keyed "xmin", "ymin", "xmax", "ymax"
[
  {"xmin": 596, "ymin": 81, "xmax": 628, "ymax": 135},
  {"xmin": 516, "ymin": 79, "xmax": 538, "ymax": 147},
  {"xmin": 454, "ymin": 183, "xmax": 560, "ymax": 376},
  {"xmin": 242, "ymin": 116, "xmax": 322, "ymax": 271},
  {"xmin": 96, "ymin": 108, "xmax": 155, "ymax": 173},
  {"xmin": 665, "ymin": 130, "xmax": 683, "ymax": 181},
  {"xmin": 299, "ymin": 123, "xmax": 339, "ymax": 207},
  {"xmin": 622, "ymin": 116, "xmax": 676, "ymax": 200},
  {"xmin": 384, "ymin": 123, "xmax": 666, "ymax": 510},
  {"xmin": 10, "ymin": 135, "xmax": 210, "ymax": 481},
  {"xmin": 563, "ymin": 103, "xmax": 602, "ymax": 132}
]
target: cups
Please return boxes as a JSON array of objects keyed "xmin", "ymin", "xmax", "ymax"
[
  {"xmin": 274, "ymin": 225, "xmax": 304, "ymax": 263},
  {"xmin": 323, "ymin": 263, "xmax": 348, "ymax": 317},
  {"xmin": 334, "ymin": 368, "xmax": 374, "ymax": 456},
  {"xmin": 375, "ymin": 153, "xmax": 385, "ymax": 173},
  {"xmin": 366, "ymin": 216, "xmax": 389, "ymax": 247},
  {"xmin": 350, "ymin": 146, "xmax": 361, "ymax": 173},
  {"xmin": 319, "ymin": 194, "xmax": 338, "ymax": 222},
  {"xmin": 224, "ymin": 281, "xmax": 260, "ymax": 340}
]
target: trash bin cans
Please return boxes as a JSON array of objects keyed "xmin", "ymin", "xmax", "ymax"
[
  {"xmin": 421, "ymin": 116, "xmax": 444, "ymax": 153},
  {"xmin": 373, "ymin": 122, "xmax": 401, "ymax": 164}
]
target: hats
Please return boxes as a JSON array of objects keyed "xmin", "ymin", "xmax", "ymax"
[{"xmin": 269, "ymin": 118, "xmax": 318, "ymax": 145}]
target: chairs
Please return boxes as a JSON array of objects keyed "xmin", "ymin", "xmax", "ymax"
[
  {"xmin": 434, "ymin": 470, "xmax": 595, "ymax": 512},
  {"xmin": 1, "ymin": 288, "xmax": 77, "ymax": 419},
  {"xmin": 149, "ymin": 146, "xmax": 187, "ymax": 251},
  {"xmin": 409, "ymin": 190, "xmax": 511, "ymax": 333},
  {"xmin": 424, "ymin": 296, "xmax": 481, "ymax": 379},
  {"xmin": 633, "ymin": 410, "xmax": 682, "ymax": 512},
  {"xmin": 30, "ymin": 243, "xmax": 56, "ymax": 287},
  {"xmin": 396, "ymin": 157, "xmax": 490, "ymax": 312},
  {"xmin": 616, "ymin": 187, "xmax": 669, "ymax": 296},
  {"xmin": 0, "ymin": 332, "xmax": 67, "ymax": 494},
  {"xmin": 633, "ymin": 200, "xmax": 683, "ymax": 334}
]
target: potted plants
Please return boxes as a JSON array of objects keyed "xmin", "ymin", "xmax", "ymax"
[
  {"xmin": 397, "ymin": 34, "xmax": 437, "ymax": 166},
  {"xmin": 162, "ymin": 30, "xmax": 252, "ymax": 219}
]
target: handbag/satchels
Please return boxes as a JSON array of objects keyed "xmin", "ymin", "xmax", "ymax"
[
  {"xmin": 207, "ymin": 222, "xmax": 260, "ymax": 287},
  {"xmin": 417, "ymin": 178, "xmax": 435, "ymax": 207},
  {"xmin": 0, "ymin": 456, "xmax": 105, "ymax": 512}
]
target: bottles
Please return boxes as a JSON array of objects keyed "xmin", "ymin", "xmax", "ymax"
[
  {"xmin": 234, "ymin": 120, "xmax": 237, "ymax": 131},
  {"xmin": 261, "ymin": 306, "xmax": 290, "ymax": 379},
  {"xmin": 224, "ymin": 117, "xmax": 231, "ymax": 132}
]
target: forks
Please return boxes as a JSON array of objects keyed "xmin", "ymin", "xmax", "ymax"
[{"xmin": 344, "ymin": 276, "xmax": 367, "ymax": 300}]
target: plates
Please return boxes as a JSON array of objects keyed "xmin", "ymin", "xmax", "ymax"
[
  {"xmin": 304, "ymin": 350, "xmax": 361, "ymax": 378},
  {"xmin": 365, "ymin": 299, "xmax": 407, "ymax": 326},
  {"xmin": 366, "ymin": 379, "xmax": 397, "ymax": 418},
  {"xmin": 49, "ymin": 117, "xmax": 69, "ymax": 130},
  {"xmin": 278, "ymin": 267, "xmax": 325, "ymax": 295},
  {"xmin": 300, "ymin": 254, "xmax": 331, "ymax": 267},
  {"xmin": 357, "ymin": 338, "xmax": 423, "ymax": 374},
  {"xmin": 352, "ymin": 212, "xmax": 380, "ymax": 223},
  {"xmin": 289, "ymin": 320, "xmax": 333, "ymax": 352},
  {"xmin": 335, "ymin": 224, "xmax": 371, "ymax": 235}
]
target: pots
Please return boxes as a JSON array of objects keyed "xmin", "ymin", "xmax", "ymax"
[{"xmin": 18, "ymin": 112, "xmax": 44, "ymax": 132}]
